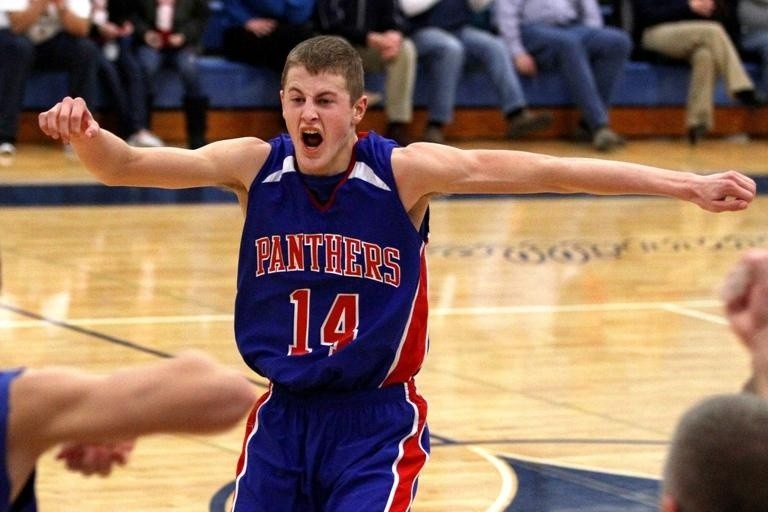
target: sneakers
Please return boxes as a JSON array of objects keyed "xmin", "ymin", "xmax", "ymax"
[
  {"xmin": 389, "ymin": 112, "xmax": 622, "ymax": 153},
  {"xmin": 1, "ymin": 123, "xmax": 165, "ymax": 158}
]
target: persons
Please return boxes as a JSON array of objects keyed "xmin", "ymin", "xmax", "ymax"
[
  {"xmin": 38, "ymin": 36, "xmax": 757, "ymax": 512},
  {"xmin": 1, "ymin": 1, "xmax": 417, "ymax": 154},
  {"xmin": 662, "ymin": 247, "xmax": 768, "ymax": 512},
  {"xmin": 399, "ymin": 1, "xmax": 768, "ymax": 151},
  {"xmin": 0, "ymin": 348, "xmax": 254, "ymax": 512}
]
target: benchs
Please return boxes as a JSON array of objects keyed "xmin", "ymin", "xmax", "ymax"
[{"xmin": 13, "ymin": 49, "xmax": 768, "ymax": 147}]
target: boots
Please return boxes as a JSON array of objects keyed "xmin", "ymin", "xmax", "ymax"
[{"xmin": 179, "ymin": 93, "xmax": 212, "ymax": 149}]
